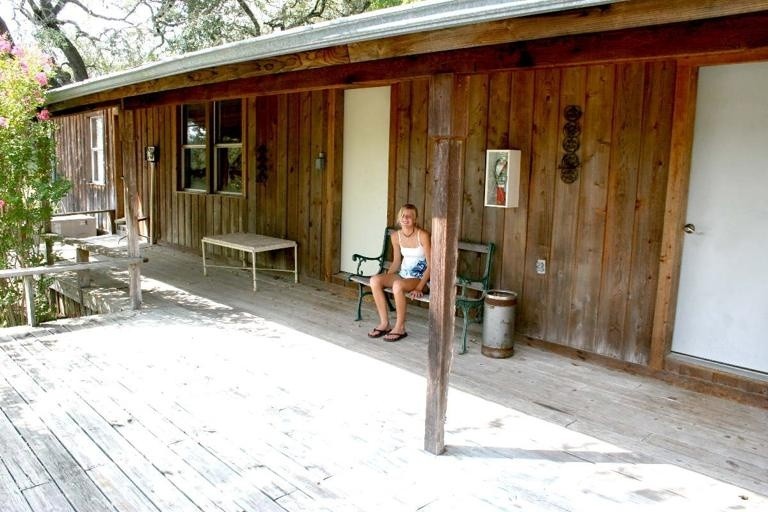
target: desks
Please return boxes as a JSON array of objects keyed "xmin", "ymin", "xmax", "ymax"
[{"xmin": 201, "ymin": 233, "xmax": 297, "ymax": 291}]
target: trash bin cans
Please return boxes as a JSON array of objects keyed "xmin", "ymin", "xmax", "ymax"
[{"xmin": 481, "ymin": 290, "xmax": 518, "ymax": 358}]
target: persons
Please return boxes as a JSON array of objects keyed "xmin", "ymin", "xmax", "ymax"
[{"xmin": 368, "ymin": 204, "xmax": 430, "ymax": 342}]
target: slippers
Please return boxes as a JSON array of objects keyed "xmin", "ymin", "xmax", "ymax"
[
  {"xmin": 384, "ymin": 331, "xmax": 407, "ymax": 341},
  {"xmin": 368, "ymin": 327, "xmax": 391, "ymax": 338}
]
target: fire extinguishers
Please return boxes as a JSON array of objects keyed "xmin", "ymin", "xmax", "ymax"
[{"xmin": 494, "ymin": 157, "xmax": 508, "ymax": 206}]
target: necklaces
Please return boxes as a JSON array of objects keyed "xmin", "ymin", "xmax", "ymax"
[{"xmin": 402, "ymin": 229, "xmax": 415, "ymax": 238}]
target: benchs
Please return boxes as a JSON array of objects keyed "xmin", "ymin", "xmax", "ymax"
[{"xmin": 344, "ymin": 227, "xmax": 496, "ymax": 354}]
